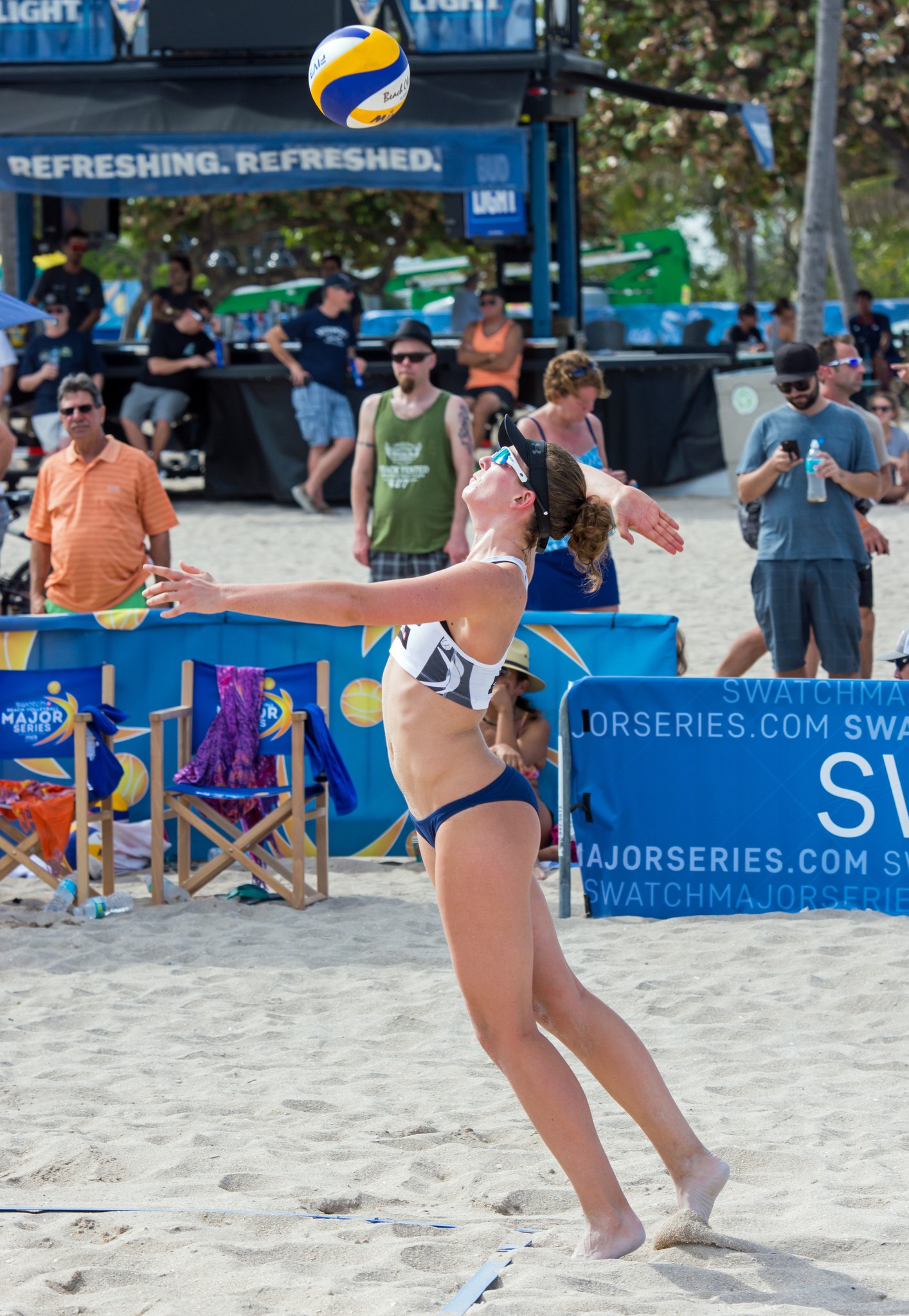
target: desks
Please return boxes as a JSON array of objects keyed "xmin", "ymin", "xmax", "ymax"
[{"xmin": 13, "ymin": 349, "xmax": 731, "ymax": 506}]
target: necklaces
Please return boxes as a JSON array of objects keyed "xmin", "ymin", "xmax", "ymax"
[{"xmin": 482, "ymin": 717, "xmax": 497, "ymax": 727}]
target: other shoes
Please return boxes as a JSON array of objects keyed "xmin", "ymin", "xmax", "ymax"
[
  {"xmin": 291, "ymin": 484, "xmax": 322, "ymax": 515},
  {"xmin": 310, "ymin": 502, "xmax": 332, "ymax": 514}
]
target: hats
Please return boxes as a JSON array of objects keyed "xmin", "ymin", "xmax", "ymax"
[
  {"xmin": 876, "ymin": 627, "xmax": 909, "ymax": 661},
  {"xmin": 503, "ymin": 637, "xmax": 546, "ymax": 693},
  {"xmin": 498, "ymin": 413, "xmax": 551, "ymax": 539},
  {"xmin": 189, "ymin": 298, "xmax": 214, "ymax": 314},
  {"xmin": 44, "ymin": 291, "xmax": 71, "ymax": 308},
  {"xmin": 325, "ymin": 273, "xmax": 357, "ymax": 290},
  {"xmin": 770, "ymin": 296, "xmax": 792, "ymax": 315},
  {"xmin": 385, "ymin": 319, "xmax": 437, "ymax": 354},
  {"xmin": 773, "ymin": 342, "xmax": 820, "ymax": 375}
]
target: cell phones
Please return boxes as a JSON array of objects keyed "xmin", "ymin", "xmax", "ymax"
[{"xmin": 781, "ymin": 439, "xmax": 800, "ymax": 461}]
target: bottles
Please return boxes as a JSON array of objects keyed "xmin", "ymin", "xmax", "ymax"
[
  {"xmin": 49, "ymin": 346, "xmax": 61, "ymax": 365},
  {"xmin": 805, "ymin": 440, "xmax": 827, "ymax": 502},
  {"xmin": 44, "ymin": 870, "xmax": 77, "ymax": 913},
  {"xmin": 73, "ymin": 891, "xmax": 134, "ymax": 919},
  {"xmin": 208, "ymin": 848, "xmax": 250, "ymax": 871},
  {"xmin": 145, "ymin": 874, "xmax": 190, "ymax": 904}
]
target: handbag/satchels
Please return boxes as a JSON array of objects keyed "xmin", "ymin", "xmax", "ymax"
[
  {"xmin": 111, "ymin": 818, "xmax": 172, "ymax": 875},
  {"xmin": 737, "ymin": 499, "xmax": 762, "ymax": 550}
]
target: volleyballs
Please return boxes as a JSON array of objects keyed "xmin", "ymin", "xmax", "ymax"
[{"xmin": 308, "ymin": 24, "xmax": 411, "ymax": 129}]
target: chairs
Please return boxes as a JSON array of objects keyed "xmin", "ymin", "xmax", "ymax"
[
  {"xmin": 0, "ymin": 661, "xmax": 117, "ymax": 914},
  {"xmin": 149, "ymin": 658, "xmax": 329, "ymax": 911}
]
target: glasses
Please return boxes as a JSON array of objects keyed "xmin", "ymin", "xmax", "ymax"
[
  {"xmin": 827, "ymin": 357, "xmax": 863, "ymax": 368},
  {"xmin": 870, "ymin": 405, "xmax": 893, "ymax": 412},
  {"xmin": 391, "ymin": 352, "xmax": 432, "ymax": 363},
  {"xmin": 71, "ymin": 244, "xmax": 87, "ymax": 251},
  {"xmin": 344, "ymin": 288, "xmax": 354, "ymax": 293},
  {"xmin": 491, "ymin": 446, "xmax": 548, "ymax": 515},
  {"xmin": 46, "ymin": 308, "xmax": 63, "ymax": 315},
  {"xmin": 896, "ymin": 655, "xmax": 909, "ymax": 671},
  {"xmin": 777, "ymin": 374, "xmax": 814, "ymax": 394},
  {"xmin": 60, "ymin": 404, "xmax": 92, "ymax": 416},
  {"xmin": 186, "ymin": 305, "xmax": 210, "ymax": 324},
  {"xmin": 480, "ymin": 300, "xmax": 496, "ymax": 306},
  {"xmin": 499, "ymin": 668, "xmax": 508, "ymax": 676},
  {"xmin": 571, "ymin": 362, "xmax": 594, "ymax": 380}
]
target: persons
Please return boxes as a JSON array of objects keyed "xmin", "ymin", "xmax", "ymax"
[
  {"xmin": 142, "ymin": 413, "xmax": 731, "ymax": 1261},
  {"xmin": 0, "ymin": 225, "xmax": 909, "ymax": 881}
]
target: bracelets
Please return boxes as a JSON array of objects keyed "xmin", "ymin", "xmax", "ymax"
[{"xmin": 859, "ymin": 520, "xmax": 870, "ymax": 530}]
